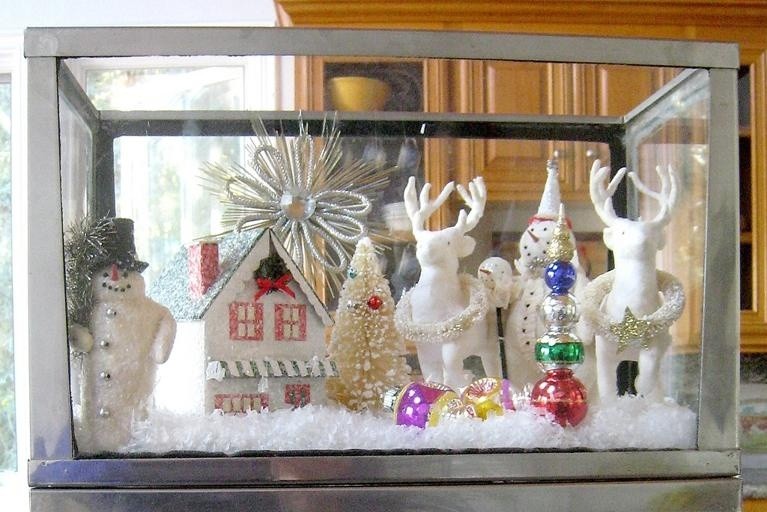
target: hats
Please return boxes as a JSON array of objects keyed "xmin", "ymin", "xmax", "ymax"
[
  {"xmin": 85, "ymin": 217, "xmax": 149, "ymax": 274},
  {"xmin": 532, "ymin": 154, "xmax": 572, "ymax": 224}
]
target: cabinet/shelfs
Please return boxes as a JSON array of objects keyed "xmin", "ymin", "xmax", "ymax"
[
  {"xmin": 451, "ymin": 2, "xmax": 676, "ymax": 197},
  {"xmin": 274, "ymin": 5, "xmax": 451, "ymax": 312},
  {"xmin": 687, "ymin": 0, "xmax": 767, "ymax": 355}
]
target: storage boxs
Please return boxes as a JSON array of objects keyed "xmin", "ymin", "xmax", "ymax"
[{"xmin": 24, "ymin": 22, "xmax": 741, "ymax": 489}]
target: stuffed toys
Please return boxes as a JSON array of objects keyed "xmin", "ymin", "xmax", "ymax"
[
  {"xmin": 505, "ymin": 162, "xmax": 594, "ymax": 419},
  {"xmin": 584, "ymin": 157, "xmax": 686, "ymax": 397},
  {"xmin": 473, "ymin": 258, "xmax": 513, "ymax": 308},
  {"xmin": 65, "ymin": 215, "xmax": 177, "ymax": 457},
  {"xmin": 392, "ymin": 174, "xmax": 512, "ymax": 395}
]
